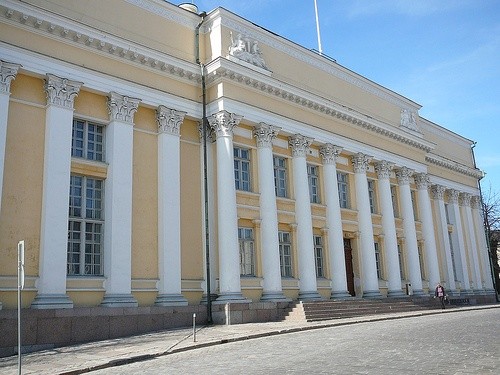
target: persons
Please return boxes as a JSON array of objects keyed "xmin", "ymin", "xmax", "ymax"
[
  {"xmin": 227, "ymin": 31, "xmax": 273, "ymax": 71},
  {"xmin": 399, "ymin": 108, "xmax": 418, "ymax": 131},
  {"xmin": 433, "ymin": 283, "xmax": 447, "ymax": 310}
]
